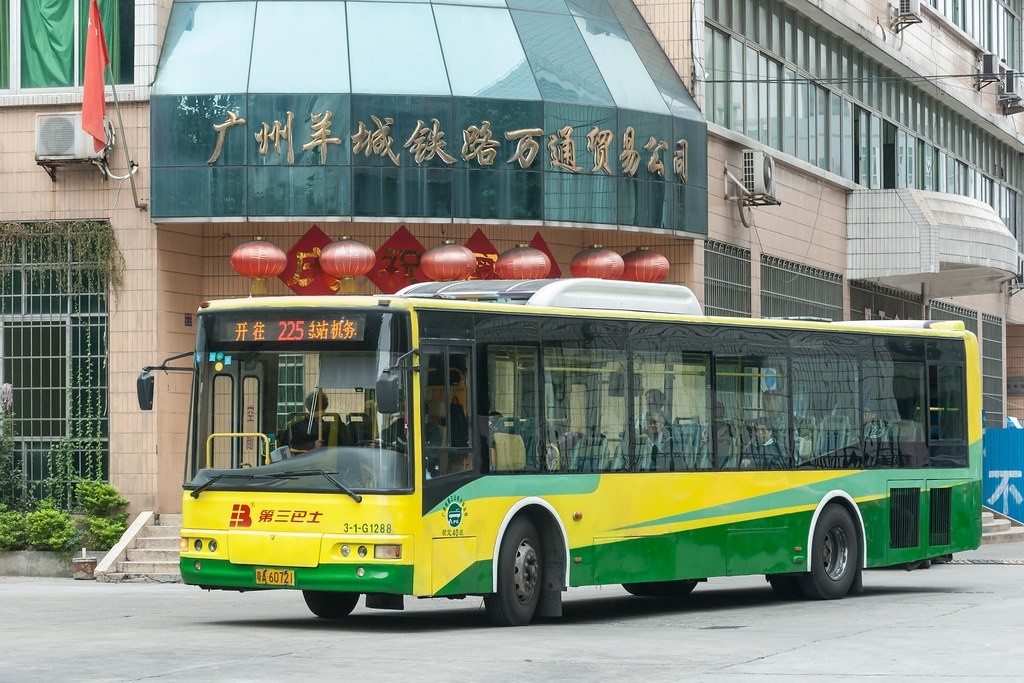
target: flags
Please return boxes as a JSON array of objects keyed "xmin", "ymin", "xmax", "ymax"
[{"xmin": 81, "ymin": 0, "xmax": 110, "ymax": 153}]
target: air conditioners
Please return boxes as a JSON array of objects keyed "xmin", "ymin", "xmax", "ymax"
[
  {"xmin": 899, "ymin": 0, "xmax": 920, "ymax": 19},
  {"xmin": 981, "ymin": 53, "xmax": 999, "ymax": 78},
  {"xmin": 1015, "ymin": 256, "xmax": 1024, "ymax": 288},
  {"xmin": 34, "ymin": 111, "xmax": 111, "ymax": 162},
  {"xmin": 1011, "ymin": 80, "xmax": 1024, "ymax": 107},
  {"xmin": 1005, "ymin": 69, "xmax": 1022, "ymax": 98},
  {"xmin": 743, "ymin": 148, "xmax": 776, "ymax": 197}
]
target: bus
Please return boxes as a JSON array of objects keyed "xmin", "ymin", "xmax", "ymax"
[{"xmin": 136, "ymin": 277, "xmax": 983, "ymax": 625}]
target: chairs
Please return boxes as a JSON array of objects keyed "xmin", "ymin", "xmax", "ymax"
[{"xmin": 290, "ymin": 391, "xmax": 966, "ymax": 471}]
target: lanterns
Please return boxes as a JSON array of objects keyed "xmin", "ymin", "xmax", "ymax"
[
  {"xmin": 230, "ymin": 236, "xmax": 287, "ymax": 294},
  {"xmin": 319, "ymin": 236, "xmax": 376, "ymax": 293},
  {"xmin": 622, "ymin": 245, "xmax": 670, "ymax": 282},
  {"xmin": 570, "ymin": 243, "xmax": 625, "ymax": 280},
  {"xmin": 420, "ymin": 239, "xmax": 476, "ymax": 281},
  {"xmin": 495, "ymin": 243, "xmax": 551, "ymax": 280}
]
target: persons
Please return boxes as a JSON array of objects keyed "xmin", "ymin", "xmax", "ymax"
[
  {"xmin": 864, "ymin": 403, "xmax": 885, "ymax": 440},
  {"xmin": 374, "ymin": 404, "xmax": 441, "ymax": 477},
  {"xmin": 636, "ymin": 389, "xmax": 688, "ymax": 469},
  {"xmin": 489, "ymin": 411, "xmax": 504, "ymax": 448},
  {"xmin": 717, "ymin": 390, "xmax": 784, "ymax": 463},
  {"xmin": 281, "ymin": 391, "xmax": 351, "ymax": 453}
]
